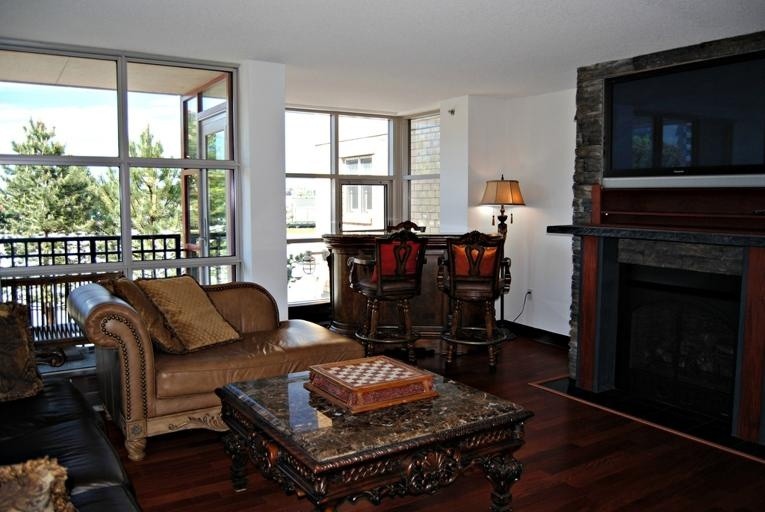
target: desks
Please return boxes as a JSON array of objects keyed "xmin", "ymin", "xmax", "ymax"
[{"xmin": 321, "ymin": 234, "xmax": 485, "ymax": 340}]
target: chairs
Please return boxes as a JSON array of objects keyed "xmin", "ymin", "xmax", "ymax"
[
  {"xmin": 347, "ymin": 220, "xmax": 430, "ymax": 367},
  {"xmin": 436, "ymin": 231, "xmax": 513, "ymax": 371}
]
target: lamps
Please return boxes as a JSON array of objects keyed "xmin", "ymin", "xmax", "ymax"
[{"xmin": 480, "ymin": 173, "xmax": 526, "ymax": 341}]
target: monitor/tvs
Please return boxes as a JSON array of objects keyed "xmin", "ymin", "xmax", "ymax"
[{"xmin": 598, "ymin": 51, "xmax": 765, "ymax": 191}]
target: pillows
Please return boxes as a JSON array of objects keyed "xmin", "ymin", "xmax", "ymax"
[
  {"xmin": 0, "ymin": 302, "xmax": 44, "ymax": 401},
  {"xmin": 0, "ymin": 455, "xmax": 80, "ymax": 511},
  {"xmin": 110, "ymin": 272, "xmax": 243, "ymax": 355}
]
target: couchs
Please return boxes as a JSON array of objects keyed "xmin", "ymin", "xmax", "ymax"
[{"xmin": 67, "ymin": 279, "xmax": 363, "ymax": 463}]
